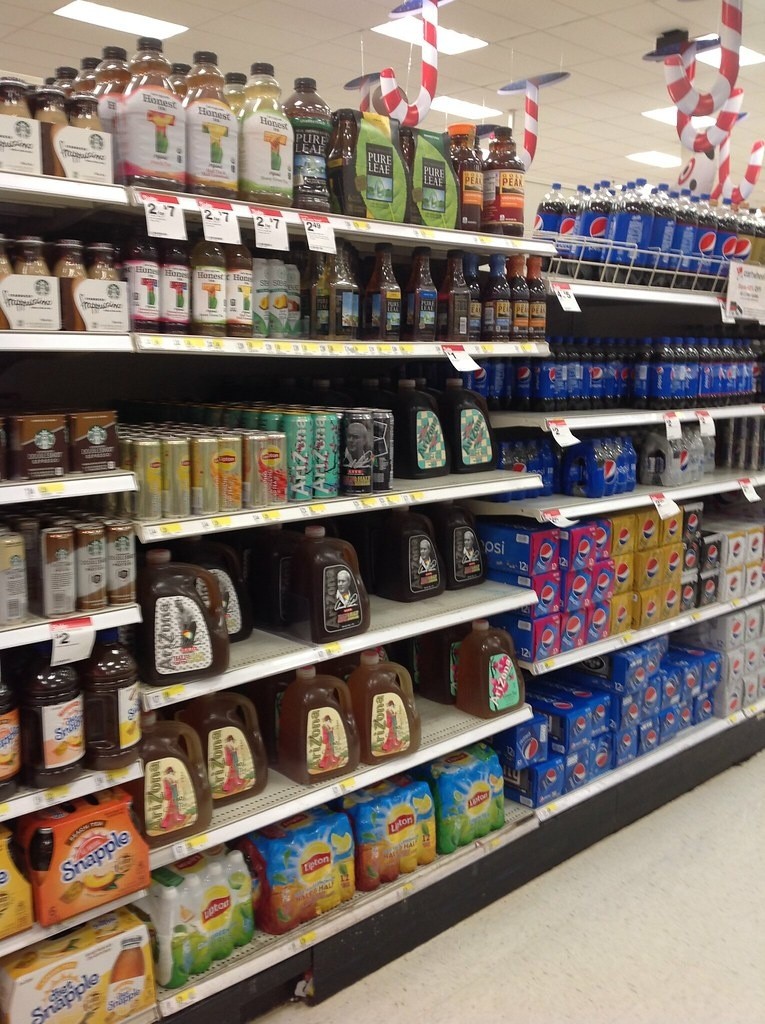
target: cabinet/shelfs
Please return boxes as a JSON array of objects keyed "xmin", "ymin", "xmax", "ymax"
[
  {"xmin": 0, "ymin": 169, "xmax": 142, "ymax": 1024},
  {"xmin": 469, "ymin": 277, "xmax": 765, "ymax": 871},
  {"xmin": 130, "ymin": 186, "xmax": 558, "ymax": 1024}
]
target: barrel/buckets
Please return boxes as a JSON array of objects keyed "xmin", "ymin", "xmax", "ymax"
[
  {"xmin": 144, "ymin": 550, "xmax": 255, "ymax": 686},
  {"xmin": 175, "ymin": 694, "xmax": 268, "ymax": 810},
  {"xmin": 131, "ymin": 711, "xmax": 212, "ymax": 848},
  {"xmin": 394, "ymin": 381, "xmax": 450, "ymax": 479},
  {"xmin": 423, "ymin": 619, "xmax": 526, "ymax": 718},
  {"xmin": 278, "ymin": 666, "xmax": 360, "ymax": 786},
  {"xmin": 291, "ymin": 525, "xmax": 370, "ymax": 644},
  {"xmin": 439, "ymin": 378, "xmax": 496, "ymax": 474},
  {"xmin": 347, "ymin": 650, "xmax": 421, "ymax": 765},
  {"xmin": 372, "ymin": 504, "xmax": 487, "ymax": 605}
]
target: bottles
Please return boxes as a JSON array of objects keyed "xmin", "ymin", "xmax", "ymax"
[
  {"xmin": 638, "ymin": 423, "xmax": 716, "ymax": 487},
  {"xmin": 252, "ymin": 258, "xmax": 301, "ymax": 338},
  {"xmin": 73, "ymin": 57, "xmax": 104, "ymax": 97},
  {"xmin": 414, "ymin": 377, "xmax": 443, "ymax": 397},
  {"xmin": 124, "ymin": 236, "xmax": 190, "ymax": 332},
  {"xmin": 98, "ymin": 38, "xmax": 191, "ymax": 192},
  {"xmin": 46, "ymin": 77, "xmax": 56, "ymax": 85},
  {"xmin": 34, "ymin": 85, "xmax": 69, "ymax": 126},
  {"xmin": 355, "ymin": 377, "xmax": 394, "ymax": 409},
  {"xmin": 485, "ymin": 253, "xmax": 528, "ymax": 341},
  {"xmin": 182, "ymin": 51, "xmax": 247, "ymax": 201},
  {"xmin": 331, "ymin": 108, "xmax": 525, "ymax": 236},
  {"xmin": 465, "ymin": 254, "xmax": 483, "ymax": 342},
  {"xmin": 86, "ymin": 629, "xmax": 140, "ymax": 772},
  {"xmin": 285, "ymin": 77, "xmax": 331, "ymax": 213},
  {"xmin": 54, "ymin": 66, "xmax": 78, "ymax": 88},
  {"xmin": 558, "ymin": 331, "xmax": 765, "ymax": 409},
  {"xmin": 18, "ymin": 658, "xmax": 85, "ymax": 791},
  {"xmin": 192, "ymin": 237, "xmax": 225, "ymax": 336},
  {"xmin": 312, "ymin": 378, "xmax": 346, "ymax": 406},
  {"xmin": 463, "ymin": 358, "xmax": 557, "ymax": 412},
  {"xmin": 237, "ymin": 63, "xmax": 294, "ymax": 207},
  {"xmin": 29, "ymin": 827, "xmax": 55, "ymax": 883},
  {"xmin": 102, "ymin": 938, "xmax": 144, "ymax": 1017},
  {"xmin": 0, "ymin": 679, "xmax": 21, "ymax": 802},
  {"xmin": 226, "ymin": 242, "xmax": 252, "ymax": 337},
  {"xmin": 555, "ymin": 435, "xmax": 639, "ymax": 497},
  {"xmin": 70, "ymin": 90, "xmax": 102, "ymax": 132},
  {"xmin": 0, "ymin": 76, "xmax": 33, "ymax": 119},
  {"xmin": 493, "ymin": 441, "xmax": 556, "ymax": 506},
  {"xmin": 0, "ymin": 231, "xmax": 119, "ymax": 281},
  {"xmin": 310, "ymin": 243, "xmax": 469, "ymax": 341},
  {"xmin": 533, "ymin": 178, "xmax": 765, "ymax": 291}
]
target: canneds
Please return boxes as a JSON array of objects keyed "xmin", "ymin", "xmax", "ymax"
[
  {"xmin": 114, "ymin": 401, "xmax": 394, "ymax": 523},
  {"xmin": 251, "ymin": 257, "xmax": 302, "ymax": 339},
  {"xmin": 0, "ymin": 504, "xmax": 137, "ymax": 626}
]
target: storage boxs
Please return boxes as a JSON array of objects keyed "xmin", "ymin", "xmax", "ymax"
[
  {"xmin": 60, "ymin": 277, "xmax": 130, "ymax": 332},
  {"xmin": 479, "ymin": 485, "xmax": 765, "ymax": 808},
  {"xmin": 0, "ymin": 273, "xmax": 62, "ymax": 330},
  {"xmin": 19, "ymin": 787, "xmax": 150, "ymax": 927},
  {"xmin": 0, "ymin": 906, "xmax": 156, "ymax": 1024}
]
